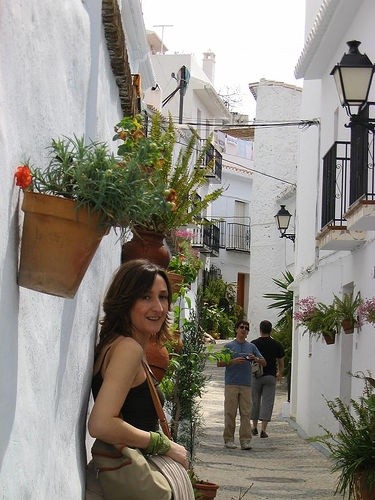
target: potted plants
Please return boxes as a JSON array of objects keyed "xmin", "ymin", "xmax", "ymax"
[
  {"xmin": 311, "ymin": 302, "xmax": 337, "ymax": 344},
  {"xmin": 178, "ymin": 336, "xmax": 219, "ymax": 500},
  {"xmin": 115, "ymin": 109, "xmax": 223, "ymax": 271},
  {"xmin": 165, "ymin": 256, "xmax": 202, "ymax": 293},
  {"xmin": 302, "ymin": 370, "xmax": 375, "ymax": 500},
  {"xmin": 323, "ymin": 291, "xmax": 361, "ymax": 334}
]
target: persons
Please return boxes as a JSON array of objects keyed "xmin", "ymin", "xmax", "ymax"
[
  {"xmin": 216, "ymin": 320, "xmax": 267, "ymax": 450},
  {"xmin": 87, "ymin": 258, "xmax": 195, "ymax": 500},
  {"xmin": 250, "ymin": 321, "xmax": 285, "ymax": 439}
]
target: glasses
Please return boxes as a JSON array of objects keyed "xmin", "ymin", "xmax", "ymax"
[{"xmin": 238, "ymin": 326, "xmax": 249, "ymax": 331}]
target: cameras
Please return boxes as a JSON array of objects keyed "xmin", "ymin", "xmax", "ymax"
[{"xmin": 244, "ymin": 354, "xmax": 252, "ymax": 361}]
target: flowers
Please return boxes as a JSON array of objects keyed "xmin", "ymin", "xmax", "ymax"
[
  {"xmin": 364, "ymin": 298, "xmax": 375, "ymax": 328},
  {"xmin": 293, "ymin": 297, "xmax": 315, "ymax": 321},
  {"xmin": 15, "ymin": 133, "xmax": 153, "ymax": 225}
]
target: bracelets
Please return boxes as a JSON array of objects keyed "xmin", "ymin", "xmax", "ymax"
[{"xmin": 143, "ymin": 431, "xmax": 171, "ymax": 457}]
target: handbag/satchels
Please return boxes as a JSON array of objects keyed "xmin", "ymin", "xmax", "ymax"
[
  {"xmin": 119, "ymin": 443, "xmax": 196, "ymax": 500},
  {"xmin": 251, "ymin": 362, "xmax": 264, "ymax": 378}
]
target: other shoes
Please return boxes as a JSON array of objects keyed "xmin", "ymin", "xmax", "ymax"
[
  {"xmin": 225, "ymin": 441, "xmax": 237, "ymax": 449},
  {"xmin": 240, "ymin": 444, "xmax": 252, "ymax": 450},
  {"xmin": 252, "ymin": 428, "xmax": 258, "ymax": 434},
  {"xmin": 260, "ymin": 431, "xmax": 268, "ymax": 437}
]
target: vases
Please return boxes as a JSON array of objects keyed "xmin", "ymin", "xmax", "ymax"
[{"xmin": 18, "ymin": 192, "xmax": 110, "ymax": 299}]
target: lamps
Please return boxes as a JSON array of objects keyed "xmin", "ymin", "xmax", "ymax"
[
  {"xmin": 330, "ymin": 41, "xmax": 375, "ymax": 121},
  {"xmin": 274, "ymin": 205, "xmax": 292, "ymax": 238}
]
